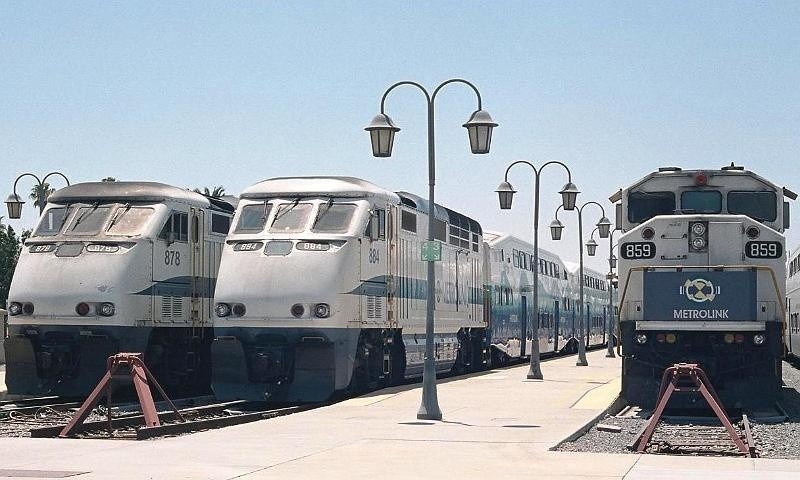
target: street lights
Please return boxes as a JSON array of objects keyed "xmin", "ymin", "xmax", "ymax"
[
  {"xmin": 366, "ymin": 79, "xmax": 497, "ymax": 420},
  {"xmin": 491, "ymin": 153, "xmax": 579, "ymax": 380}
]
